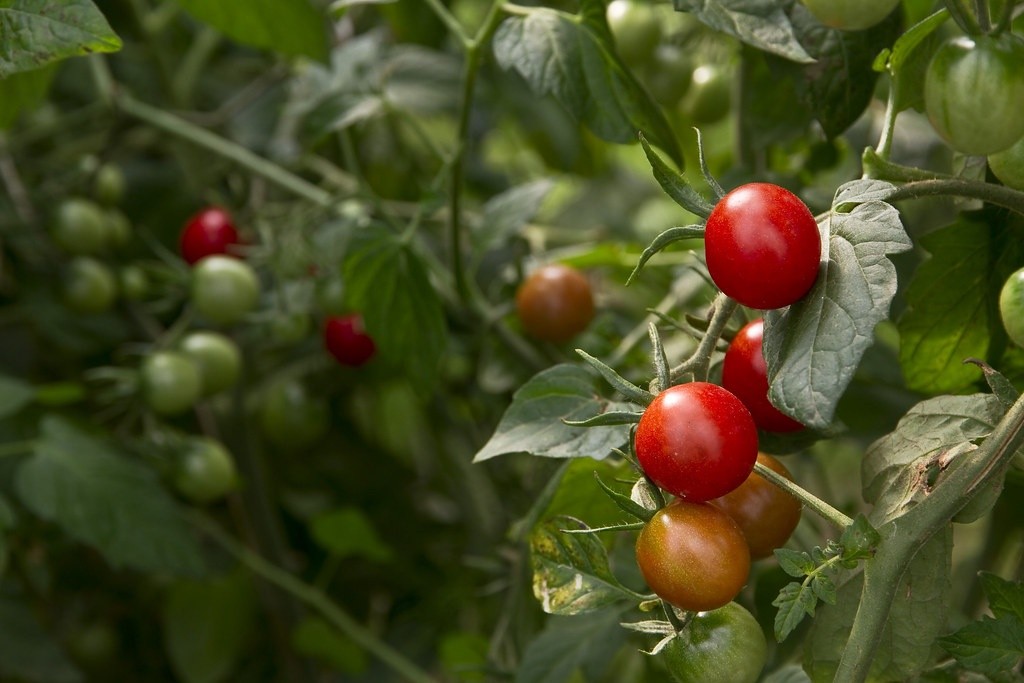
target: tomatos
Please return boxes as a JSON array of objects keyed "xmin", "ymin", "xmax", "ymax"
[
  {"xmin": 57, "ymin": 150, "xmax": 376, "ymax": 506},
  {"xmin": 634, "ymin": 317, "xmax": 803, "ymax": 611},
  {"xmin": 515, "ymin": 267, "xmax": 597, "ymax": 343},
  {"xmin": 922, "ymin": 31, "xmax": 1024, "ymax": 191},
  {"xmin": 999, "ymin": 267, "xmax": 1024, "ymax": 345},
  {"xmin": 802, "ymin": 2, "xmax": 905, "ymax": 30},
  {"xmin": 601, "ymin": 0, "xmax": 732, "ymax": 125},
  {"xmin": 706, "ymin": 179, "xmax": 823, "ymax": 310}
]
